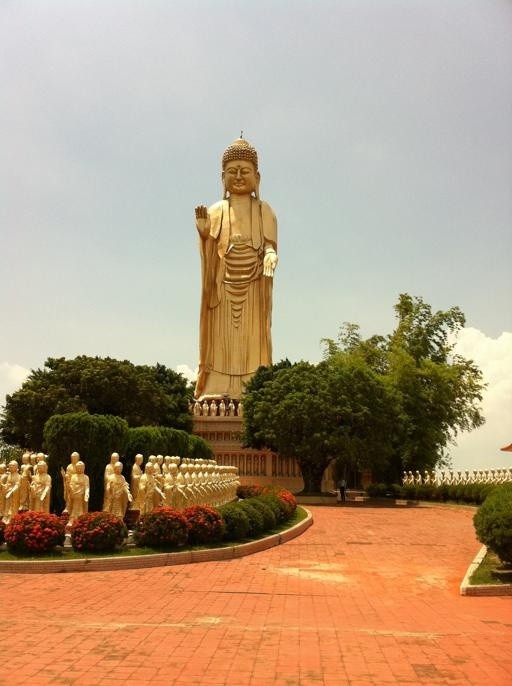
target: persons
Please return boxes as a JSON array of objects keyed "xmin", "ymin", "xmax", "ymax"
[
  {"xmin": 337, "ymin": 476, "xmax": 346, "ymax": 502},
  {"xmin": 187, "ymin": 398, "xmax": 244, "ymax": 416},
  {"xmin": 195, "ymin": 139, "xmax": 278, "ymax": 396},
  {"xmin": 0, "ymin": 452, "xmax": 241, "ymax": 529}
]
